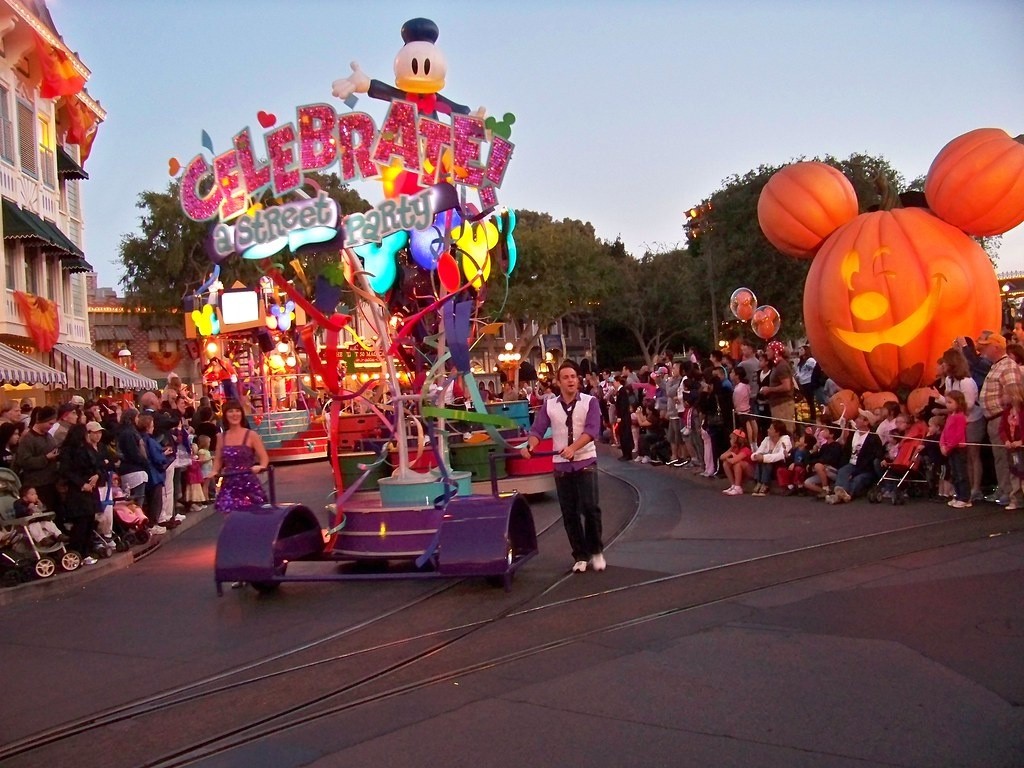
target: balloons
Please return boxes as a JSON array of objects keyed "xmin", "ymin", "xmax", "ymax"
[
  {"xmin": 730, "ymin": 288, "xmax": 757, "ymax": 323},
  {"xmin": 751, "ymin": 305, "xmax": 780, "ymax": 341}
]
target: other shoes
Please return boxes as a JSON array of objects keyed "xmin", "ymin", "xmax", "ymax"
[
  {"xmin": 190, "ymin": 503, "xmax": 207, "ymax": 512},
  {"xmin": 590, "ymin": 552, "xmax": 607, "ymax": 572},
  {"xmin": 174, "ymin": 513, "xmax": 186, "ymax": 522},
  {"xmin": 985, "ymin": 494, "xmax": 1010, "ymax": 505},
  {"xmin": 158, "ymin": 519, "xmax": 182, "ymax": 530},
  {"xmin": 1005, "ymin": 504, "xmax": 1024, "ymax": 510},
  {"xmin": 929, "ymin": 494, "xmax": 952, "ymax": 503},
  {"xmin": 572, "ymin": 560, "xmax": 588, "ymax": 573},
  {"xmin": 231, "ymin": 582, "xmax": 247, "ymax": 588},
  {"xmin": 606, "ymin": 441, "xmax": 851, "ymax": 505},
  {"xmin": 970, "ymin": 490, "xmax": 983, "ymax": 502},
  {"xmin": 947, "ymin": 499, "xmax": 972, "ymax": 508},
  {"xmin": 147, "ymin": 525, "xmax": 166, "ymax": 535}
]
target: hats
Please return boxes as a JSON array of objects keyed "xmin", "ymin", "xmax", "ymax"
[
  {"xmin": 768, "ymin": 340, "xmax": 786, "ymax": 357},
  {"xmin": 979, "ymin": 334, "xmax": 1007, "ymax": 350},
  {"xmin": 20, "ymin": 397, "xmax": 33, "ymax": 410},
  {"xmin": 858, "ymin": 407, "xmax": 877, "ymax": 427},
  {"xmin": 655, "ymin": 367, "xmax": 668, "ymax": 374},
  {"xmin": 85, "ymin": 421, "xmax": 106, "ymax": 432},
  {"xmin": 69, "ymin": 395, "xmax": 85, "ymax": 410},
  {"xmin": 733, "ymin": 429, "xmax": 747, "ymax": 439}
]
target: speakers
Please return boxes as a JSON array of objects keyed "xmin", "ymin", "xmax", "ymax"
[{"xmin": 257, "ymin": 330, "xmax": 275, "ymax": 352}]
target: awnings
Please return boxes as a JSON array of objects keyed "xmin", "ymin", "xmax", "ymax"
[
  {"xmin": 2, "ymin": 197, "xmax": 94, "ymax": 274},
  {"xmin": 56, "ymin": 144, "xmax": 89, "ymax": 181},
  {"xmin": 51, "ymin": 344, "xmax": 158, "ymax": 392},
  {"xmin": 0, "ymin": 342, "xmax": 68, "ymax": 386}
]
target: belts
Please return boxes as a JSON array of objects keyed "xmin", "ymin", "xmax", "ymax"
[{"xmin": 987, "ymin": 411, "xmax": 1002, "ymax": 422}]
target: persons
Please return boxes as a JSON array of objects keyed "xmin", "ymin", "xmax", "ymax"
[
  {"xmin": 268, "ymin": 355, "xmax": 297, "ymax": 411},
  {"xmin": 203, "ymin": 357, "xmax": 240, "ymax": 402},
  {"xmin": 521, "ymin": 364, "xmax": 606, "ymax": 574},
  {"xmin": 0, "ymin": 372, "xmax": 222, "ymax": 565},
  {"xmin": 316, "ymin": 391, "xmax": 416, "ymax": 425},
  {"xmin": 423, "ymin": 362, "xmax": 473, "ymax": 446},
  {"xmin": 483, "ymin": 296, "xmax": 1024, "ymax": 509},
  {"xmin": 203, "ymin": 400, "xmax": 268, "ymax": 588}
]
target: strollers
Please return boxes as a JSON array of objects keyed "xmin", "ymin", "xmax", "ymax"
[
  {"xmin": 0, "ymin": 468, "xmax": 80, "ymax": 581},
  {"xmin": 87, "ymin": 468, "xmax": 150, "ymax": 558},
  {"xmin": 868, "ymin": 422, "xmax": 935, "ymax": 505}
]
